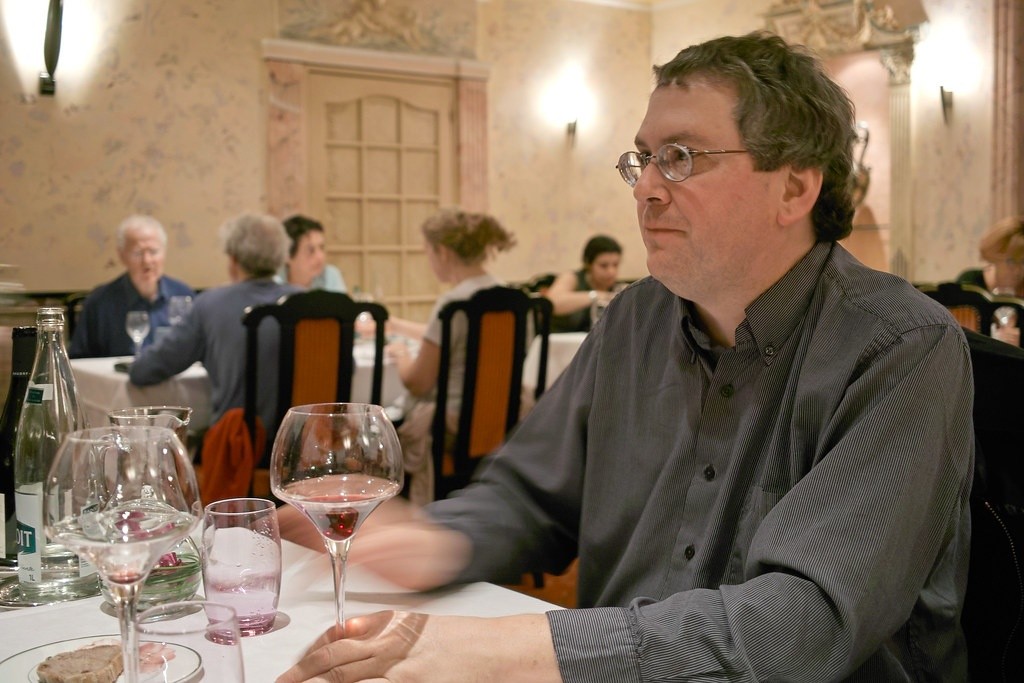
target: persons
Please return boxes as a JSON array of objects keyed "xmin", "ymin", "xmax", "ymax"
[
  {"xmin": 129, "ymin": 213, "xmax": 309, "ymax": 469},
  {"xmin": 269, "ymin": 29, "xmax": 977, "ymax": 683},
  {"xmin": 540, "ymin": 235, "xmax": 635, "ymax": 334},
  {"xmin": 354, "ymin": 208, "xmax": 536, "ymax": 475},
  {"xmin": 65, "ymin": 215, "xmax": 197, "ymax": 362},
  {"xmin": 277, "ymin": 216, "xmax": 348, "ymax": 295},
  {"xmin": 973, "ymin": 218, "xmax": 1024, "ymax": 349}
]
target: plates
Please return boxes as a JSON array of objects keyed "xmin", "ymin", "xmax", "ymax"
[{"xmin": 0, "ymin": 633, "xmax": 202, "ymax": 683}]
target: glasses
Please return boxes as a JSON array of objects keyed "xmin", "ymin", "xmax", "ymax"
[{"xmin": 615, "ymin": 150, "xmax": 773, "ymax": 189}]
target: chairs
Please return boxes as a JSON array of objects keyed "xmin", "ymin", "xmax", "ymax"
[
  {"xmin": 408, "ymin": 284, "xmax": 553, "ymax": 498},
  {"xmin": 914, "ymin": 281, "xmax": 1024, "ymax": 349},
  {"xmin": 186, "ymin": 289, "xmax": 389, "ymax": 502}
]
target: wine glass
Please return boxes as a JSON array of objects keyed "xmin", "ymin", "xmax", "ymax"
[
  {"xmin": 44, "ymin": 426, "xmax": 201, "ymax": 683},
  {"xmin": 125, "ymin": 310, "xmax": 151, "ymax": 364},
  {"xmin": 270, "ymin": 402, "xmax": 405, "ymax": 643}
]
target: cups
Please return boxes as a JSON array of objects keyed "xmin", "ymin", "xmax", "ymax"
[
  {"xmin": 202, "ymin": 497, "xmax": 282, "ymax": 638},
  {"xmin": 352, "ymin": 292, "xmax": 371, "ymax": 324},
  {"xmin": 169, "ymin": 296, "xmax": 192, "ymax": 325},
  {"xmin": 131, "ymin": 600, "xmax": 245, "ymax": 683}
]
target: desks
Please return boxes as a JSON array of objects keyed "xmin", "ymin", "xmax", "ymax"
[{"xmin": 68, "ymin": 345, "xmax": 399, "ymax": 454}]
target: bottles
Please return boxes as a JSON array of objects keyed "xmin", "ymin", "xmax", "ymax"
[{"xmin": 0, "ymin": 306, "xmax": 102, "ymax": 604}]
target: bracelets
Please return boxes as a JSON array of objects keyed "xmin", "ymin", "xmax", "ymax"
[{"xmin": 589, "ymin": 290, "xmax": 599, "ymax": 304}]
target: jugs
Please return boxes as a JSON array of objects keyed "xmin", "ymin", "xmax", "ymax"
[{"xmin": 97, "ymin": 405, "xmax": 201, "ymax": 616}]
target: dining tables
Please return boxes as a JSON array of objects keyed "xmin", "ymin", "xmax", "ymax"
[{"xmin": 1, "ymin": 482, "xmax": 569, "ymax": 683}]
